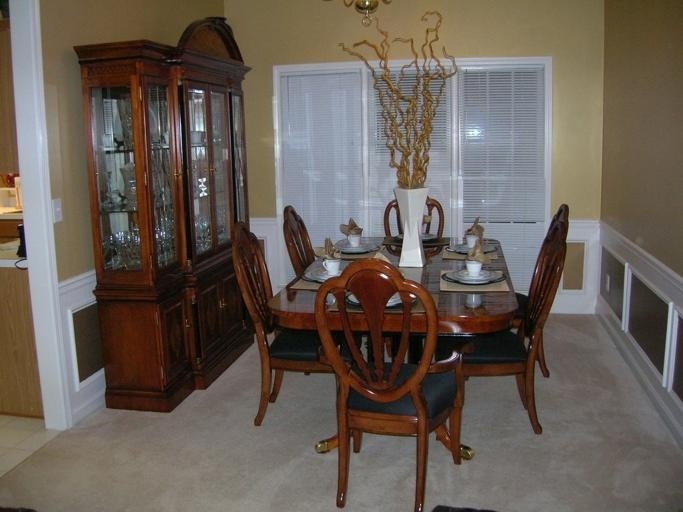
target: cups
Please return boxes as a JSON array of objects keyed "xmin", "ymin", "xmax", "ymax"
[
  {"xmin": 464, "ymin": 260, "xmax": 482, "ymax": 276},
  {"xmin": 346, "ymin": 233, "xmax": 360, "ymax": 248},
  {"xmin": 465, "ymin": 234, "xmax": 478, "ymax": 248},
  {"xmin": 320, "ymin": 258, "xmax": 341, "ymax": 277},
  {"xmin": 464, "ymin": 292, "xmax": 482, "ymax": 309},
  {"xmin": 103, "ymin": 98, "xmax": 227, "ymax": 268}
]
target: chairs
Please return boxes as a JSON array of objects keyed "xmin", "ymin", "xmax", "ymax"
[
  {"xmin": 312, "ymin": 256, "xmax": 465, "ymax": 512},
  {"xmin": 230, "ymin": 218, "xmax": 352, "ymax": 428},
  {"xmin": 283, "ymin": 202, "xmax": 316, "ymax": 272},
  {"xmin": 433, "ymin": 221, "xmax": 569, "ymax": 442},
  {"xmin": 509, "ymin": 202, "xmax": 569, "ymax": 385},
  {"xmin": 383, "ymin": 194, "xmax": 445, "ymax": 240}
]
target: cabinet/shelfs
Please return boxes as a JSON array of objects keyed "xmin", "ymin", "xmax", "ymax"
[{"xmin": 73, "ymin": 17, "xmax": 261, "ymax": 413}]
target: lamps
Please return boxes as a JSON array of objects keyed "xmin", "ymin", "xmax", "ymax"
[{"xmin": 340, "ymin": 1, "xmax": 394, "ymax": 26}]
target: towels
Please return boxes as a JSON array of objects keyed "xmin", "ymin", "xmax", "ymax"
[
  {"xmin": 465, "ymin": 239, "xmax": 491, "ymax": 264},
  {"xmin": 372, "ymin": 250, "xmax": 405, "ymax": 277},
  {"xmin": 337, "ymin": 217, "xmax": 363, "ymax": 235},
  {"xmin": 313, "ymin": 239, "xmax": 342, "ymax": 258},
  {"xmin": 463, "ymin": 215, "xmax": 486, "ymax": 236},
  {"xmin": 422, "ymin": 212, "xmax": 432, "ymax": 226}
]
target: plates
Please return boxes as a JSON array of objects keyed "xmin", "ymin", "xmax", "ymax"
[
  {"xmin": 333, "ymin": 238, "xmax": 379, "ymax": 254},
  {"xmin": 445, "ymin": 240, "xmax": 498, "ymax": 256},
  {"xmin": 397, "ymin": 233, "xmax": 436, "ymax": 241},
  {"xmin": 438, "ymin": 268, "xmax": 506, "ymax": 285},
  {"xmin": 344, "ymin": 292, "xmax": 406, "ymax": 309},
  {"xmin": 300, "ymin": 266, "xmax": 332, "ymax": 284}
]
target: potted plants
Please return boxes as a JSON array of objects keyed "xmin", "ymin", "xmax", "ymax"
[{"xmin": 339, "ymin": 7, "xmax": 457, "ymax": 266}]
